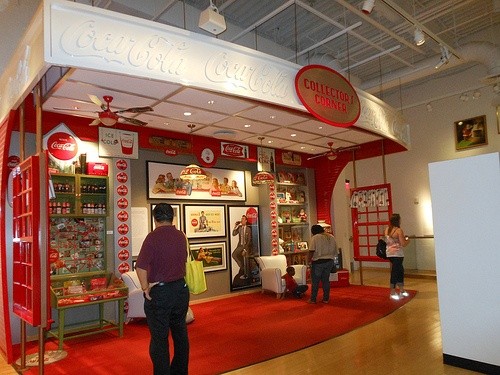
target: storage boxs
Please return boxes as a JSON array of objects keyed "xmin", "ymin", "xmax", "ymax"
[
  {"xmin": 86, "ymin": 162, "xmax": 108, "ymax": 176},
  {"xmin": 319, "ymin": 270, "xmax": 349, "ymax": 287}
]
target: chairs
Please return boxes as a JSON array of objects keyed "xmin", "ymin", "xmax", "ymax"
[{"xmin": 257, "ymin": 255, "xmax": 306, "ymax": 299}]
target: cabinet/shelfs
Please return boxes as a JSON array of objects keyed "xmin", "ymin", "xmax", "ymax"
[
  {"xmin": 49, "ymin": 172, "xmax": 109, "ymax": 217},
  {"xmin": 259, "ymin": 182, "xmax": 311, "ymax": 265},
  {"xmin": 12, "ymin": 155, "xmax": 40, "ymax": 327}
]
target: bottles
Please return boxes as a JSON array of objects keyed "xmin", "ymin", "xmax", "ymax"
[
  {"xmin": 236, "ymin": 221, "xmax": 241, "ymax": 225},
  {"xmin": 50, "ymin": 178, "xmax": 107, "ymax": 214}
]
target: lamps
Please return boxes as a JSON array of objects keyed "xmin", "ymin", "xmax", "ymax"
[
  {"xmin": 252, "ymin": 137, "xmax": 275, "ymax": 183},
  {"xmin": 327, "ymin": 153, "xmax": 337, "ymax": 160},
  {"xmin": 440, "ymin": 47, "xmax": 448, "ymax": 64},
  {"xmin": 362, "ymin": 0, "xmax": 375, "ymax": 15},
  {"xmin": 180, "ymin": 124, "xmax": 207, "ymax": 181},
  {"xmin": 98, "ymin": 112, "xmax": 119, "ymax": 126},
  {"xmin": 414, "ymin": 29, "xmax": 425, "ymax": 45}
]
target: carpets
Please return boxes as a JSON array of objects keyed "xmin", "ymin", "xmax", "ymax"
[{"xmin": 0, "ymin": 283, "xmax": 418, "ymax": 375}]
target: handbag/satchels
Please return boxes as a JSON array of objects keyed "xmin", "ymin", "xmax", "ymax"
[
  {"xmin": 330, "ymin": 264, "xmax": 337, "ymax": 273},
  {"xmin": 376, "ymin": 239, "xmax": 387, "ymax": 259},
  {"xmin": 182, "ymin": 232, "xmax": 208, "ymax": 294}
]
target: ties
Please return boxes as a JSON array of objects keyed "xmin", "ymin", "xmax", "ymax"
[{"xmin": 243, "ymin": 227, "xmax": 244, "ymax": 244}]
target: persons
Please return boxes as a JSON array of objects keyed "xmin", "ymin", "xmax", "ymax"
[
  {"xmin": 209, "ymin": 178, "xmax": 242, "ymax": 197},
  {"xmin": 279, "ymin": 267, "xmax": 309, "ymax": 301},
  {"xmin": 385, "ymin": 214, "xmax": 410, "ymax": 300},
  {"xmin": 134, "ymin": 203, "xmax": 190, "ymax": 375},
  {"xmin": 199, "ymin": 211, "xmax": 206, "ymax": 229},
  {"xmin": 197, "ymin": 248, "xmax": 222, "ymax": 263},
  {"xmin": 232, "ymin": 215, "xmax": 252, "ymax": 279},
  {"xmin": 306, "ymin": 225, "xmax": 336, "ymax": 304},
  {"xmin": 152, "ymin": 172, "xmax": 183, "ymax": 193}
]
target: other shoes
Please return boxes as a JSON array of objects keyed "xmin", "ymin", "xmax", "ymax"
[
  {"xmin": 390, "ymin": 293, "xmax": 400, "ymax": 300},
  {"xmin": 318, "ymin": 300, "xmax": 329, "ymax": 303},
  {"xmin": 240, "ymin": 275, "xmax": 248, "ymax": 279},
  {"xmin": 308, "ymin": 300, "xmax": 316, "ymax": 304},
  {"xmin": 400, "ymin": 291, "xmax": 410, "ymax": 296}
]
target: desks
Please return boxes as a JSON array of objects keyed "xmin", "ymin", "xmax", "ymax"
[{"xmin": 48, "ymin": 280, "xmax": 128, "ymax": 351}]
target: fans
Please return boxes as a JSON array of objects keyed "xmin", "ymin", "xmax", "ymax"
[
  {"xmin": 53, "ymin": 94, "xmax": 154, "ymax": 126},
  {"xmin": 307, "ymin": 142, "xmax": 360, "ymax": 161}
]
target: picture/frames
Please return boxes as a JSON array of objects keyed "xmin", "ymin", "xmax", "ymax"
[
  {"xmin": 182, "ymin": 204, "xmax": 227, "ymax": 240},
  {"xmin": 453, "ymin": 115, "xmax": 488, "ymax": 150},
  {"xmin": 150, "ymin": 203, "xmax": 181, "ymax": 232},
  {"xmin": 227, "ymin": 205, "xmax": 262, "ymax": 292},
  {"xmin": 145, "ymin": 160, "xmax": 246, "ymax": 202},
  {"xmin": 188, "ymin": 241, "xmax": 228, "ymax": 273}
]
